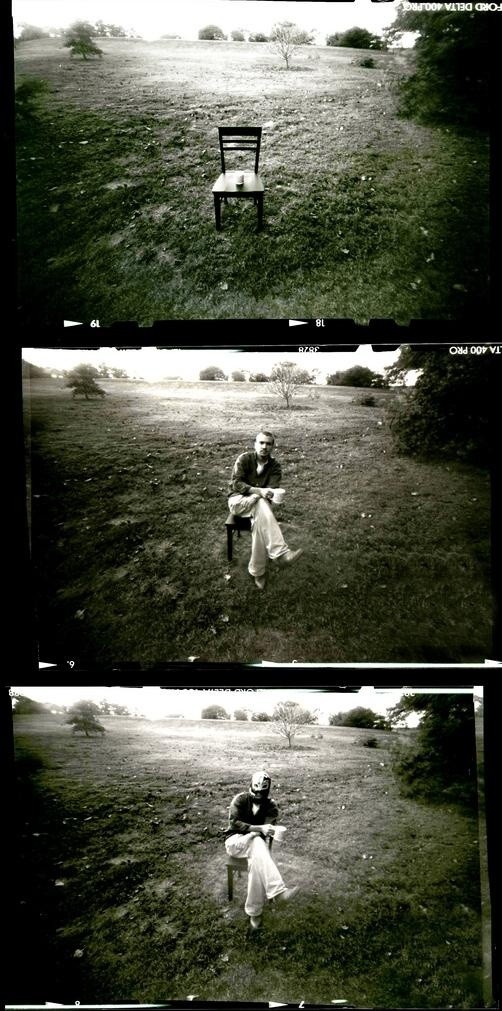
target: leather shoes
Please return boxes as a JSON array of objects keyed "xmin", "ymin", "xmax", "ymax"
[
  {"xmin": 273, "ymin": 547, "xmax": 304, "ymax": 571},
  {"xmin": 250, "ymin": 914, "xmax": 263, "ymax": 929},
  {"xmin": 276, "ymin": 886, "xmax": 301, "ymax": 905},
  {"xmin": 254, "ymin": 572, "xmax": 266, "ymax": 589}
]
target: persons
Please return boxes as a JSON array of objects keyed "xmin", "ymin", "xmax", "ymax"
[
  {"xmin": 224, "ymin": 770, "xmax": 300, "ymax": 944},
  {"xmin": 225, "ymin": 430, "xmax": 304, "ymax": 591}
]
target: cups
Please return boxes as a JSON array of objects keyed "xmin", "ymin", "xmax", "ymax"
[
  {"xmin": 269, "ymin": 488, "xmax": 285, "ymax": 505},
  {"xmin": 234, "ymin": 171, "xmax": 244, "ymax": 186},
  {"xmin": 270, "ymin": 826, "xmax": 287, "ymax": 843}
]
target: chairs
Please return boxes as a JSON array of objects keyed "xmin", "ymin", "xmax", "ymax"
[
  {"xmin": 211, "ymin": 125, "xmax": 264, "ymax": 233},
  {"xmin": 225, "ymin": 818, "xmax": 278, "ymax": 906}
]
